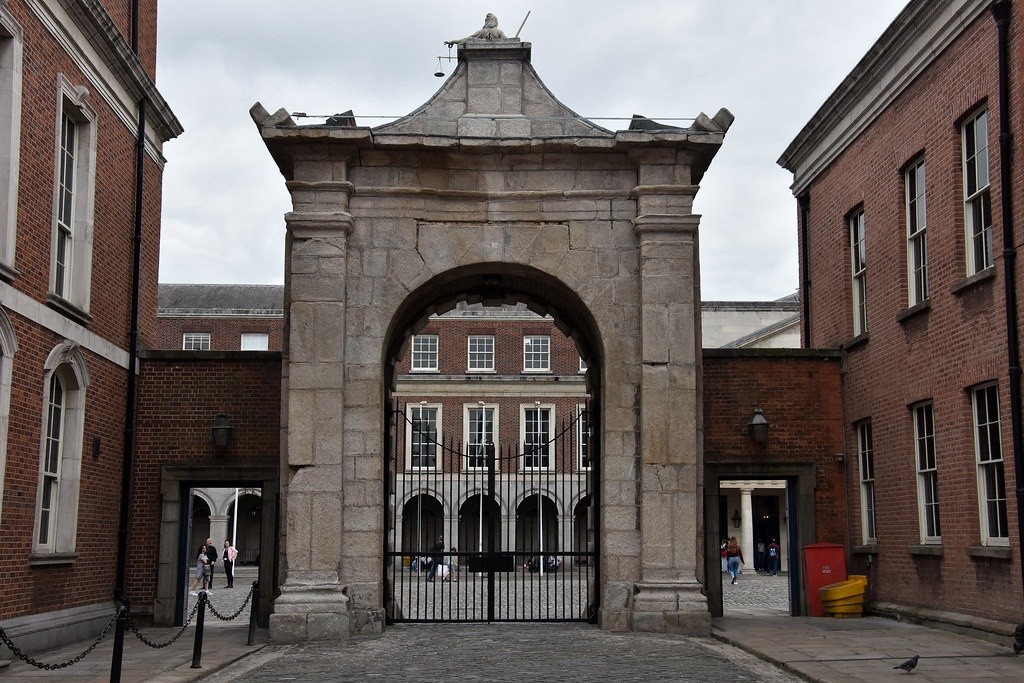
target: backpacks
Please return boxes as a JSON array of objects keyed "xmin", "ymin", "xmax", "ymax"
[{"xmin": 769, "ymin": 547, "xmax": 776, "ymax": 557}]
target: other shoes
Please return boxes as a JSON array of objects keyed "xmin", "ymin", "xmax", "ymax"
[
  {"xmin": 204, "ymin": 590, "xmax": 214, "ymax": 596},
  {"xmin": 762, "ymin": 568, "xmax": 764, "ymax": 572},
  {"xmin": 734, "ymin": 581, "xmax": 738, "ymax": 585},
  {"xmin": 428, "ymin": 577, "xmax": 435, "ymax": 582},
  {"xmin": 224, "ymin": 585, "xmax": 233, "ymax": 588},
  {"xmin": 199, "ymin": 586, "xmax": 205, "ymax": 589},
  {"xmin": 208, "ymin": 586, "xmax": 211, "ymax": 589},
  {"xmin": 767, "ymin": 572, "xmax": 770, "ymax": 576},
  {"xmin": 189, "ymin": 591, "xmax": 198, "ymax": 597},
  {"xmin": 446, "ymin": 579, "xmax": 450, "ymax": 582},
  {"xmin": 731, "ymin": 576, "xmax": 735, "ymax": 584},
  {"xmin": 453, "ymin": 578, "xmax": 458, "ymax": 582},
  {"xmin": 758, "ymin": 567, "xmax": 761, "ymax": 572},
  {"xmin": 773, "ymin": 573, "xmax": 777, "ymax": 576}
]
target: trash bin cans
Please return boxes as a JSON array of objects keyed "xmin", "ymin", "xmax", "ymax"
[
  {"xmin": 801, "ymin": 542, "xmax": 847, "ymax": 616},
  {"xmin": 404, "ymin": 556, "xmax": 410, "ymax": 567}
]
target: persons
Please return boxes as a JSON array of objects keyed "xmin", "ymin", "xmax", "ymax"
[
  {"xmin": 721, "ymin": 536, "xmax": 780, "ymax": 585},
  {"xmin": 221, "ymin": 540, "xmax": 239, "ymax": 588},
  {"xmin": 527, "ymin": 553, "xmax": 563, "ymax": 572},
  {"xmin": 412, "ymin": 536, "xmax": 460, "ymax": 582},
  {"xmin": 190, "ymin": 538, "xmax": 219, "ymax": 596},
  {"xmin": 443, "ymin": 13, "xmax": 508, "ymax": 47}
]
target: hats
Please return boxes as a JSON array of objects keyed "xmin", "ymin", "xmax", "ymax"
[{"xmin": 773, "ymin": 538, "xmax": 776, "ymax": 541}]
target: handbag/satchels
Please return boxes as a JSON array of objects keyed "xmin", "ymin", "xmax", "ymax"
[
  {"xmin": 202, "ymin": 564, "xmax": 212, "ymax": 576},
  {"xmin": 438, "ymin": 563, "xmax": 449, "ymax": 577}
]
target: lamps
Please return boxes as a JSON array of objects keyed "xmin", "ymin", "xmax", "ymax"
[
  {"xmin": 745, "ymin": 405, "xmax": 771, "ymax": 446},
  {"xmin": 732, "ymin": 508, "xmax": 742, "ymax": 527},
  {"xmin": 209, "ymin": 412, "xmax": 235, "ymax": 449}
]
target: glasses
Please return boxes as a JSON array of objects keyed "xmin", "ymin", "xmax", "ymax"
[{"xmin": 209, "ymin": 542, "xmax": 212, "ymax": 544}]
